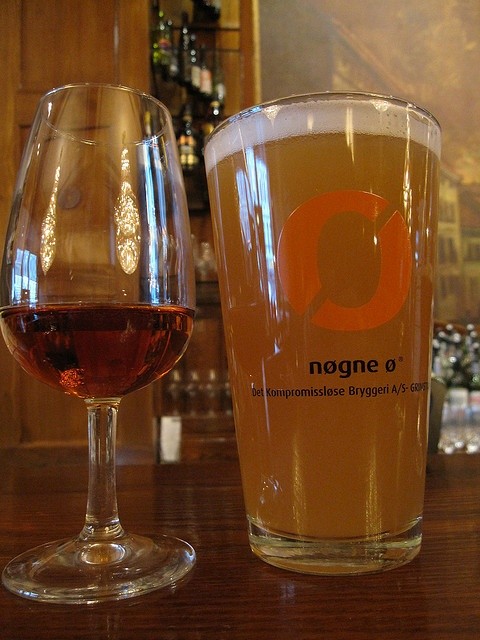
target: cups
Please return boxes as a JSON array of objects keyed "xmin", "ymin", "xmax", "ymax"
[{"xmin": 201, "ymin": 93, "xmax": 441, "ymax": 578}]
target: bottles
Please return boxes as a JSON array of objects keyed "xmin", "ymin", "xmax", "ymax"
[
  {"xmin": 182, "ymin": 101, "xmax": 198, "ymax": 175},
  {"xmin": 205, "ymin": 99, "xmax": 221, "ymax": 141},
  {"xmin": 183, "ymin": 368, "xmax": 199, "ymax": 413},
  {"xmin": 150, "ymin": 6, "xmax": 218, "ymax": 91},
  {"xmin": 166, "ymin": 369, "xmax": 180, "ymax": 417},
  {"xmin": 203, "ymin": 366, "xmax": 219, "ymax": 414},
  {"xmin": 434, "ymin": 319, "xmax": 480, "ymax": 409},
  {"xmin": 143, "ymin": 108, "xmax": 155, "ymax": 146},
  {"xmin": 221, "ymin": 362, "xmax": 233, "ymax": 416}
]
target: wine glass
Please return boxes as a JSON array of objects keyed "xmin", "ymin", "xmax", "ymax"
[{"xmin": 0, "ymin": 83, "xmax": 198, "ymax": 602}]
